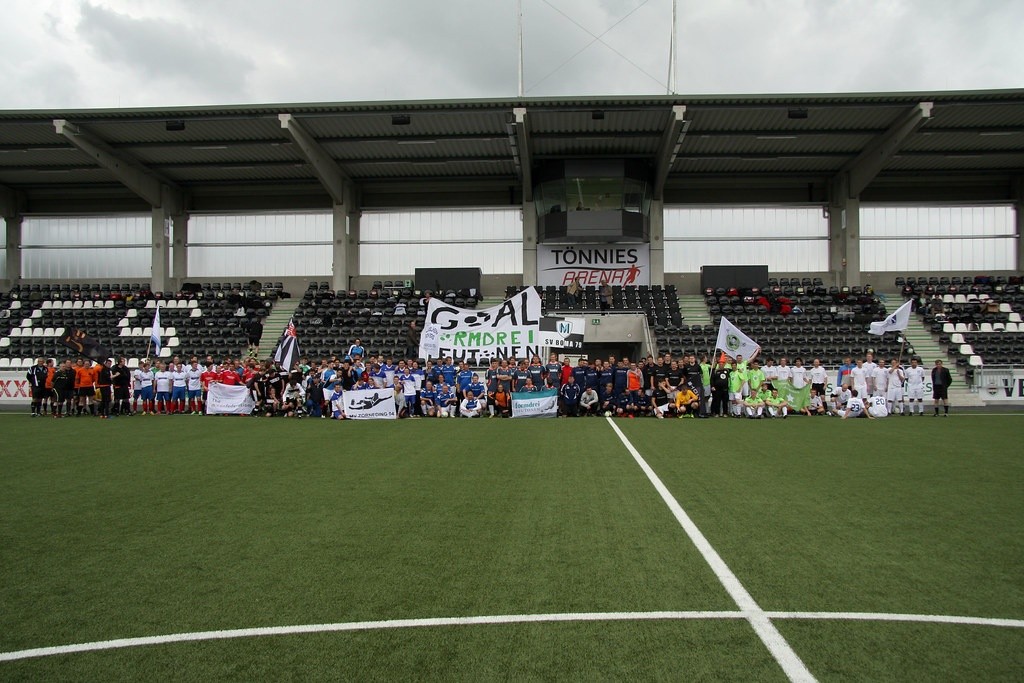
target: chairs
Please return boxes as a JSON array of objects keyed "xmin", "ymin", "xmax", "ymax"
[
  {"xmin": 506, "ymin": 276, "xmax": 1024, "ymax": 369},
  {"xmin": 0, "ymin": 281, "xmax": 283, "ymax": 370},
  {"xmin": 294, "ymin": 281, "xmax": 477, "ymax": 366}
]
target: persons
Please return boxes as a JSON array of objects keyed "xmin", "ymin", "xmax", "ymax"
[
  {"xmin": 932, "ymin": 359, "xmax": 953, "ymax": 417},
  {"xmin": 26, "ymin": 279, "xmax": 925, "ymax": 419}
]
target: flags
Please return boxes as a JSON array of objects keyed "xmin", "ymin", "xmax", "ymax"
[
  {"xmin": 151, "ymin": 302, "xmax": 162, "ymax": 356},
  {"xmin": 274, "ymin": 318, "xmax": 301, "ymax": 373},
  {"xmin": 717, "ymin": 316, "xmax": 759, "ymax": 361},
  {"xmin": 868, "ymin": 299, "xmax": 913, "ymax": 335}
]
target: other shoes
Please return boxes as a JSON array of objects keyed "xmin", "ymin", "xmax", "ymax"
[
  {"xmin": 749, "ymin": 414, "xmax": 753, "ymax": 418},
  {"xmin": 934, "ymin": 413, "xmax": 939, "ymax": 417},
  {"xmin": 772, "ymin": 414, "xmax": 776, "ymax": 417},
  {"xmin": 489, "ymin": 414, "xmax": 494, "ymax": 419},
  {"xmin": 782, "ymin": 414, "xmax": 788, "ymax": 419},
  {"xmin": 920, "ymin": 412, "xmax": 923, "ymax": 415},
  {"xmin": 758, "ymin": 415, "xmax": 762, "ymax": 419},
  {"xmin": 909, "ymin": 413, "xmax": 914, "ymax": 416},
  {"xmin": 944, "ymin": 413, "xmax": 948, "ymax": 417},
  {"xmin": 715, "ymin": 414, "xmax": 718, "ymax": 418},
  {"xmin": 31, "ymin": 411, "xmax": 204, "ymax": 418},
  {"xmin": 901, "ymin": 412, "xmax": 905, "ymax": 416},
  {"xmin": 724, "ymin": 415, "xmax": 728, "ymax": 417}
]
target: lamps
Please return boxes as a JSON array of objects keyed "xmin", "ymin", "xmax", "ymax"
[
  {"xmin": 391, "ymin": 115, "xmax": 411, "ymax": 125},
  {"xmin": 591, "ymin": 111, "xmax": 605, "ymax": 120},
  {"xmin": 166, "ymin": 120, "xmax": 186, "ymax": 131},
  {"xmin": 787, "ymin": 109, "xmax": 809, "ymax": 119}
]
